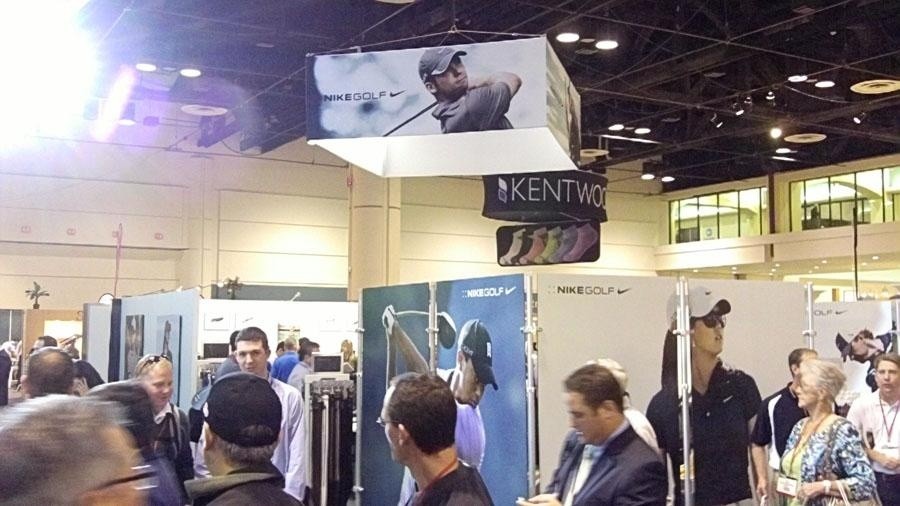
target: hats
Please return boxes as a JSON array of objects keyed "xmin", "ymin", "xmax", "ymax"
[
  {"xmin": 667, "ymin": 286, "xmax": 731, "ymax": 332},
  {"xmin": 458, "ymin": 319, "xmax": 498, "ymax": 389},
  {"xmin": 836, "ymin": 325, "xmax": 866, "ymax": 362},
  {"xmin": 419, "ymin": 47, "xmax": 467, "ymax": 81},
  {"xmin": 192, "ymin": 373, "xmax": 282, "ymax": 448}
]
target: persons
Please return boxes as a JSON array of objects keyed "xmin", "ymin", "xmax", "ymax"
[
  {"xmin": 374, "ymin": 372, "xmax": 495, "ymax": 506},
  {"xmin": 779, "ymin": 358, "xmax": 876, "ymax": 505},
  {"xmin": 382, "ymin": 304, "xmax": 498, "ymax": 506},
  {"xmin": 515, "ymin": 365, "xmax": 669, "ymax": 506},
  {"xmin": 846, "ymin": 353, "xmax": 900, "ymax": 505},
  {"xmin": 835, "ymin": 308, "xmax": 897, "ymax": 411},
  {"xmin": 419, "ymin": 48, "xmax": 523, "ymax": 133},
  {"xmin": 645, "ymin": 284, "xmax": 772, "ymax": 505},
  {"xmin": 0, "ymin": 324, "xmax": 355, "ymax": 506},
  {"xmin": 558, "ymin": 359, "xmax": 659, "ymax": 462},
  {"xmin": 752, "ymin": 349, "xmax": 840, "ymax": 505}
]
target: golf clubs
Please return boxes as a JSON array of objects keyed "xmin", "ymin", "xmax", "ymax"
[{"xmin": 385, "ymin": 310, "xmax": 458, "ymax": 350}]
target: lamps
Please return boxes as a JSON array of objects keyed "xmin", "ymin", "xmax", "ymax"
[
  {"xmin": 119, "ymin": 102, "xmax": 136, "ymax": 126},
  {"xmin": 709, "ymin": 89, "xmax": 776, "ymax": 129},
  {"xmin": 641, "ymin": 163, "xmax": 675, "ymax": 184}
]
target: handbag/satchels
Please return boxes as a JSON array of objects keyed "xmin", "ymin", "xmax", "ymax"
[{"xmin": 818, "ymin": 479, "xmax": 882, "ymax": 506}]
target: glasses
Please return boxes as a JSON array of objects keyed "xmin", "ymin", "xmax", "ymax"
[
  {"xmin": 136, "ymin": 353, "xmax": 172, "ymax": 378},
  {"xmin": 702, "ymin": 311, "xmax": 726, "ymax": 328},
  {"xmin": 376, "ymin": 417, "xmax": 400, "ymax": 428},
  {"xmin": 88, "ymin": 464, "xmax": 158, "ymax": 492}
]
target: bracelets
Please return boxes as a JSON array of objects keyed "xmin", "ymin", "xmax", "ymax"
[{"xmin": 821, "ymin": 479, "xmax": 831, "ymax": 496}]
